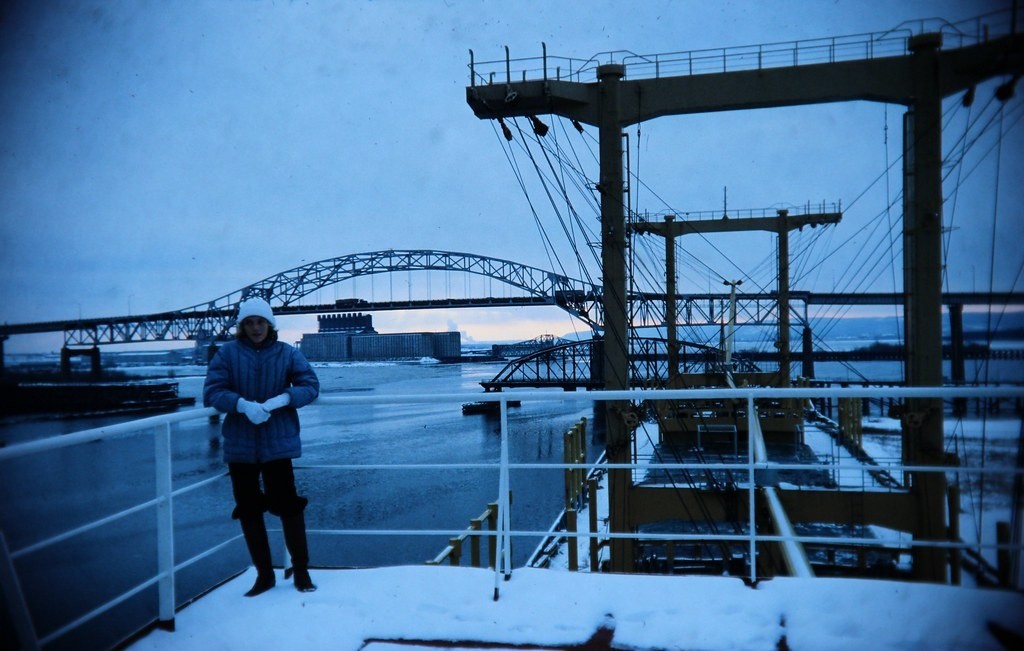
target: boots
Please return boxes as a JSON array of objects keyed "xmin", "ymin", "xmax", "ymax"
[
  {"xmin": 282, "ymin": 511, "xmax": 315, "ymax": 592},
  {"xmin": 239, "ymin": 513, "xmax": 276, "ymax": 598}
]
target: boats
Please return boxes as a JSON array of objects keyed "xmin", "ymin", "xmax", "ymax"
[
  {"xmin": 462, "ymin": 399, "xmax": 520, "ymax": 411},
  {"xmin": 419, "ymin": 356, "xmax": 440, "ymax": 365}
]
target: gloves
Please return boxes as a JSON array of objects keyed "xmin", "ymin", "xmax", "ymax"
[
  {"xmin": 264, "ymin": 392, "xmax": 291, "ymax": 411},
  {"xmin": 236, "ymin": 397, "xmax": 272, "ymax": 424}
]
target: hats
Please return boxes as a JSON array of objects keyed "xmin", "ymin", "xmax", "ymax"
[{"xmin": 236, "ymin": 297, "xmax": 276, "ymax": 328}]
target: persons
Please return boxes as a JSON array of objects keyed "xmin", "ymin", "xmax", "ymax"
[{"xmin": 203, "ymin": 296, "xmax": 321, "ymax": 597}]
